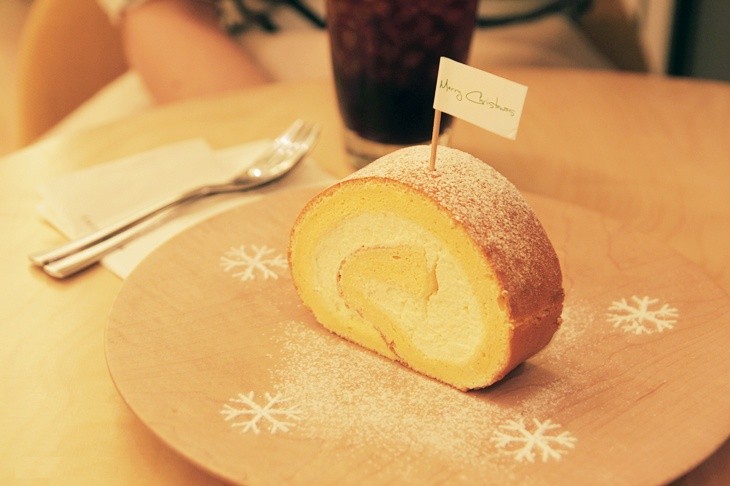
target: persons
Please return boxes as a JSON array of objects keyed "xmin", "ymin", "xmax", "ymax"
[{"xmin": 26, "ymin": 0, "xmax": 622, "ymax": 142}]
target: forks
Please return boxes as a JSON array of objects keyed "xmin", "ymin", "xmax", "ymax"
[{"xmin": 31, "ymin": 119, "xmax": 329, "ymax": 279}]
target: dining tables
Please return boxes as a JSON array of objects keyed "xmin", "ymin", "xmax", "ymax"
[{"xmin": 0, "ymin": 25, "xmax": 729, "ymax": 486}]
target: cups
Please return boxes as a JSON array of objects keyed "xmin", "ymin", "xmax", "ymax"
[{"xmin": 326, "ymin": 0, "xmax": 481, "ymax": 170}]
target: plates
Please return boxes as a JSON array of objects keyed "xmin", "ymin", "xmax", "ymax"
[{"xmin": 101, "ymin": 177, "xmax": 730, "ymax": 484}]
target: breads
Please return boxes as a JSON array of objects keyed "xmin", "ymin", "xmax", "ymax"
[{"xmin": 287, "ymin": 144, "xmax": 565, "ymax": 392}]
